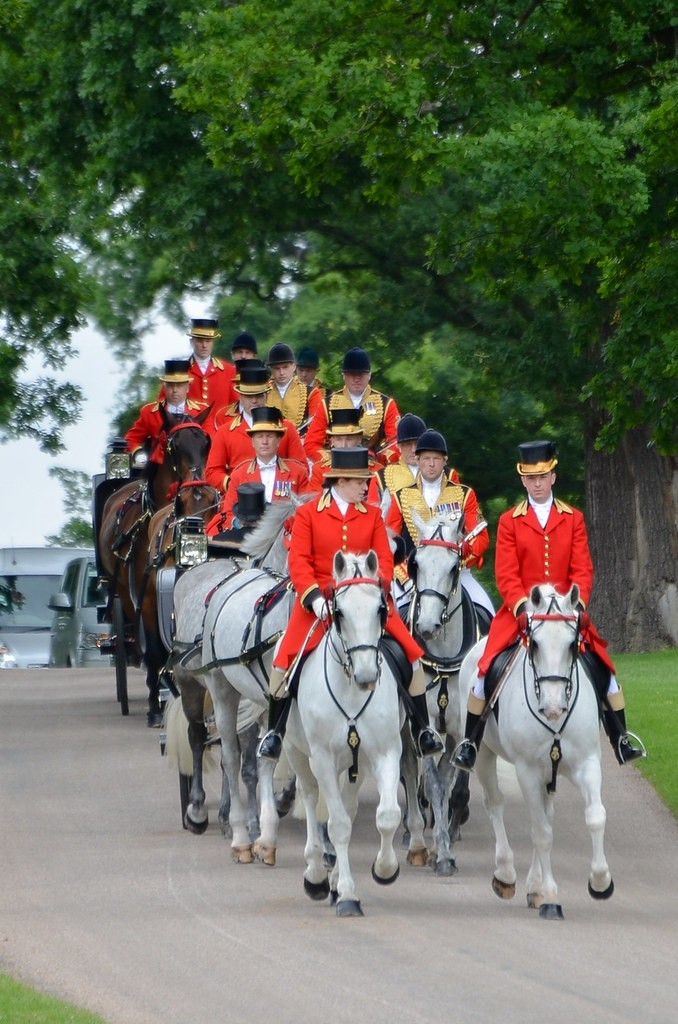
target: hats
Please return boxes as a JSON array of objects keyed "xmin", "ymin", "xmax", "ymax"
[
  {"xmin": 232, "ymin": 334, "xmax": 258, "ymax": 354},
  {"xmin": 295, "ymin": 347, "xmax": 319, "ymax": 368},
  {"xmin": 234, "ymin": 369, "xmax": 273, "ymax": 395},
  {"xmin": 232, "ymin": 483, "xmax": 271, "ymax": 520},
  {"xmin": 231, "ymin": 359, "xmax": 265, "ymax": 381},
  {"xmin": 323, "ymin": 448, "xmax": 376, "ymax": 478},
  {"xmin": 158, "ymin": 360, "xmax": 194, "ymax": 383},
  {"xmin": 517, "ymin": 441, "xmax": 557, "ymax": 475},
  {"xmin": 187, "ymin": 319, "xmax": 222, "ymax": 338},
  {"xmin": 267, "ymin": 343, "xmax": 295, "ymax": 364},
  {"xmin": 414, "ymin": 430, "xmax": 448, "ymax": 456},
  {"xmin": 326, "ymin": 409, "xmax": 364, "ymax": 435},
  {"xmin": 397, "ymin": 414, "xmax": 427, "ymax": 443},
  {"xmin": 245, "ymin": 407, "xmax": 286, "ymax": 433},
  {"xmin": 341, "ymin": 347, "xmax": 370, "ymax": 372}
]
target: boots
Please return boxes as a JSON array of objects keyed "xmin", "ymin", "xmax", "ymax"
[
  {"xmin": 456, "ymin": 712, "xmax": 486, "ymax": 772},
  {"xmin": 407, "ymin": 695, "xmax": 442, "ymax": 755},
  {"xmin": 602, "ymin": 685, "xmax": 641, "ymax": 764},
  {"xmin": 259, "ymin": 700, "xmax": 292, "ymax": 757}
]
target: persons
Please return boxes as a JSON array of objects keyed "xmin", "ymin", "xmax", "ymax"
[
  {"xmin": 123, "ymin": 316, "xmax": 490, "ymax": 598},
  {"xmin": 455, "ymin": 440, "xmax": 643, "ymax": 769},
  {"xmin": 260, "ymin": 446, "xmax": 444, "ymax": 758}
]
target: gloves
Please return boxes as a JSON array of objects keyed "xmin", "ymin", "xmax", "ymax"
[
  {"xmin": 312, "ymin": 597, "xmax": 330, "ymax": 620},
  {"xmin": 135, "ymin": 455, "xmax": 147, "ymax": 463}
]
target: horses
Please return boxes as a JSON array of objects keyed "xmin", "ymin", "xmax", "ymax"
[{"xmin": 95, "ymin": 402, "xmax": 617, "ymax": 924}]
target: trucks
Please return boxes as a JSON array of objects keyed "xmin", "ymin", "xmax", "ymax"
[{"xmin": 0, "ymin": 547, "xmax": 96, "ymax": 670}]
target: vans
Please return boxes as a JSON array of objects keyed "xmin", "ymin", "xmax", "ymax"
[{"xmin": 48, "ymin": 557, "xmax": 115, "ymax": 668}]
